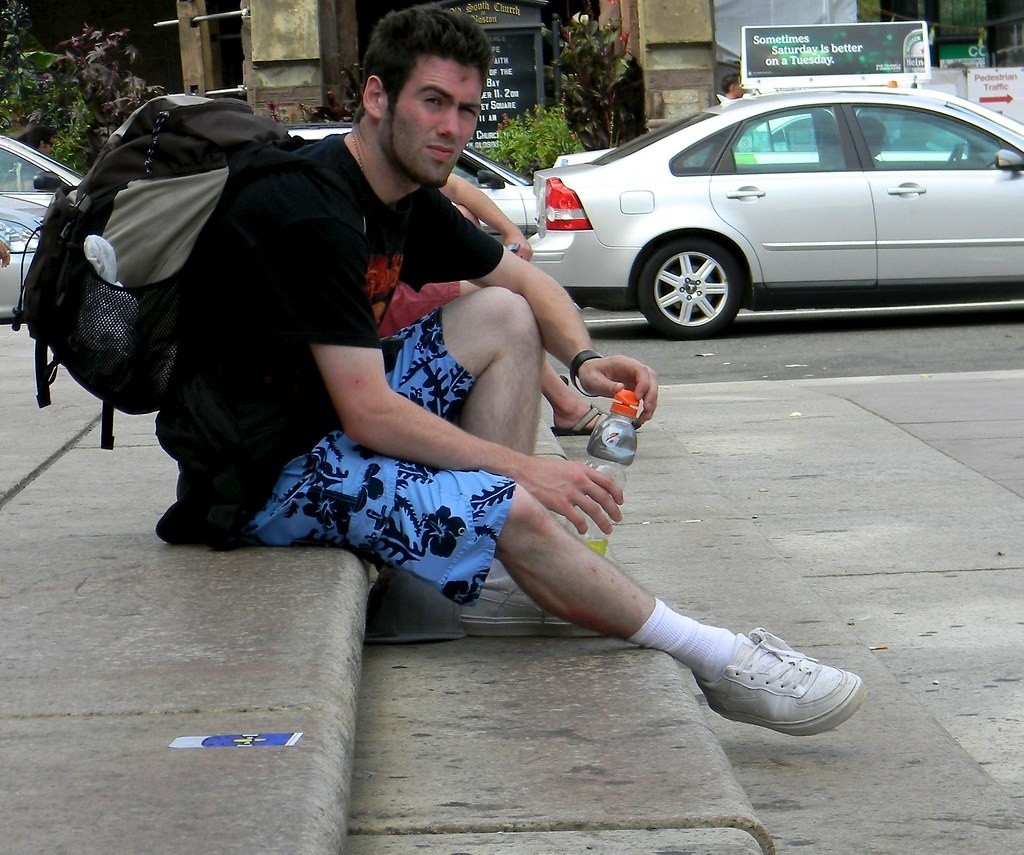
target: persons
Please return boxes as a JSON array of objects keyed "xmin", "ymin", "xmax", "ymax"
[
  {"xmin": 720, "ymin": 74, "xmax": 745, "ymax": 100},
  {"xmin": 856, "ymin": 114, "xmax": 886, "ymax": 164},
  {"xmin": 435, "ymin": 170, "xmax": 645, "ymax": 440},
  {"xmin": 156, "ymin": 6, "xmax": 867, "ymax": 739}
]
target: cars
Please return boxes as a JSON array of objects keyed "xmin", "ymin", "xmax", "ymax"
[
  {"xmin": 528, "ymin": 88, "xmax": 1024, "ymax": 342},
  {"xmin": 1, "ymin": 132, "xmax": 86, "ymax": 322}
]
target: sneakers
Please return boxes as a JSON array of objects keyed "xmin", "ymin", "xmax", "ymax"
[
  {"xmin": 692, "ymin": 627, "xmax": 868, "ymax": 736},
  {"xmin": 459, "ymin": 562, "xmax": 615, "ymax": 633}
]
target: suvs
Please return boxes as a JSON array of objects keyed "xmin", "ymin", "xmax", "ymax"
[{"xmin": 281, "ymin": 120, "xmax": 539, "ymax": 245}]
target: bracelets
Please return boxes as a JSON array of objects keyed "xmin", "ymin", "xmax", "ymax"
[{"xmin": 569, "ymin": 348, "xmax": 605, "ymax": 398}]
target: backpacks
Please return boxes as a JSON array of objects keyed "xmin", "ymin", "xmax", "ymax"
[{"xmin": 12, "ymin": 93, "xmax": 367, "ymax": 451}]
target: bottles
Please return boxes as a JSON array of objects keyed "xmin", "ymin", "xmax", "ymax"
[{"xmin": 567, "ymin": 387, "xmax": 643, "ymax": 557}]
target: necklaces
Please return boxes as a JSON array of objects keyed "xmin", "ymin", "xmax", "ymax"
[{"xmin": 350, "ymin": 132, "xmax": 370, "ymax": 186}]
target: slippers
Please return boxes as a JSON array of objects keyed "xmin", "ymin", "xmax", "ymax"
[{"xmin": 550, "ymin": 403, "xmax": 643, "ymax": 436}]
